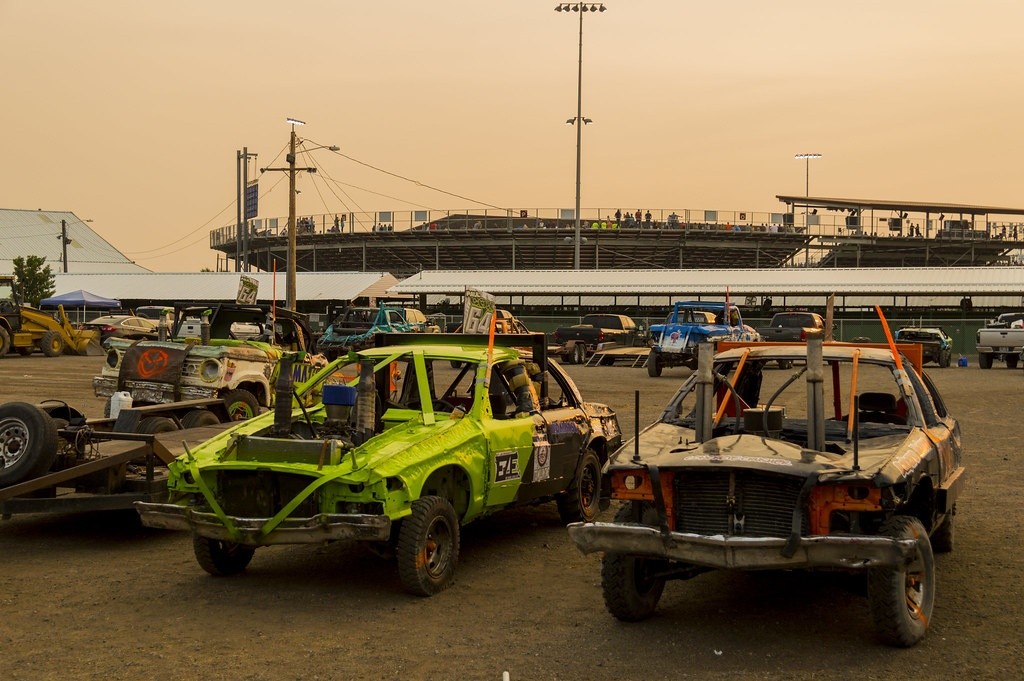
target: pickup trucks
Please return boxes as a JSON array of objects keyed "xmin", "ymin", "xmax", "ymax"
[
  {"xmin": 92, "ymin": 304, "xmax": 348, "ymax": 419},
  {"xmin": 755, "ymin": 311, "xmax": 838, "ymax": 341},
  {"xmin": 975, "ymin": 312, "xmax": 1024, "ymax": 369},
  {"xmin": 556, "ymin": 313, "xmax": 645, "ymax": 362}
]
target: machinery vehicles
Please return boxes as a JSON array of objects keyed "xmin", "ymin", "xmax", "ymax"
[{"xmin": 0, "ymin": 274, "xmax": 107, "ymax": 358}]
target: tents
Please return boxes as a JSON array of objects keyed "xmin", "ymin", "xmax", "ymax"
[{"xmin": 39, "ymin": 289, "xmax": 121, "ymax": 329}]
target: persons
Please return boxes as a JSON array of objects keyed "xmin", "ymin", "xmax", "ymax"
[
  {"xmin": 910, "ymin": 223, "xmax": 914, "ymax": 236},
  {"xmin": 693, "ymin": 222, "xmax": 795, "ymax": 233},
  {"xmin": 715, "ymin": 310, "xmax": 724, "ymax": 325},
  {"xmin": 915, "ymin": 224, "xmax": 921, "ymax": 236},
  {"xmin": 1013, "ymin": 226, "xmax": 1017, "ymax": 241},
  {"xmin": 372, "ymin": 223, "xmax": 392, "ymax": 231},
  {"xmin": 1001, "ymin": 226, "xmax": 1006, "ymax": 238},
  {"xmin": 730, "ymin": 310, "xmax": 740, "ymax": 327},
  {"xmin": 583, "ymin": 209, "xmax": 685, "ymax": 229},
  {"xmin": 538, "ymin": 218, "xmax": 574, "ymax": 228},
  {"xmin": 334, "ymin": 217, "xmax": 339, "ymax": 228},
  {"xmin": 331, "ymin": 226, "xmax": 336, "ymax": 232},
  {"xmin": 341, "ymin": 218, "xmax": 344, "ymax": 232}
]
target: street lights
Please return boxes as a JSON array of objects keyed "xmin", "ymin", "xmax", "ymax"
[
  {"xmin": 554, "ymin": 2, "xmax": 608, "ymax": 270},
  {"xmin": 286, "ymin": 116, "xmax": 307, "ymax": 311},
  {"xmin": 795, "ymin": 152, "xmax": 822, "ymax": 268}
]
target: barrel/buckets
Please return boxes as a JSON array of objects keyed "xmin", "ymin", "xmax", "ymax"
[{"xmin": 958, "ymin": 357, "xmax": 967, "ymax": 367}]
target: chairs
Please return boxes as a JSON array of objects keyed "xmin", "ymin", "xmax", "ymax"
[{"xmin": 843, "ymin": 392, "xmax": 907, "ymax": 425}]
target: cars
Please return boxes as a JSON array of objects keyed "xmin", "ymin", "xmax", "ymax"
[
  {"xmin": 565, "ymin": 339, "xmax": 966, "ymax": 649},
  {"xmin": 664, "ymin": 311, "xmax": 717, "ymax": 324},
  {"xmin": 895, "ymin": 326, "xmax": 953, "ymax": 367},
  {"xmin": 130, "ymin": 331, "xmax": 622, "ymax": 598},
  {"xmin": 79, "ymin": 313, "xmax": 171, "ymax": 343}
]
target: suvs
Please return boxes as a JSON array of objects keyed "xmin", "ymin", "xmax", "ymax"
[{"xmin": 647, "ymin": 301, "xmax": 761, "ymax": 376}]
